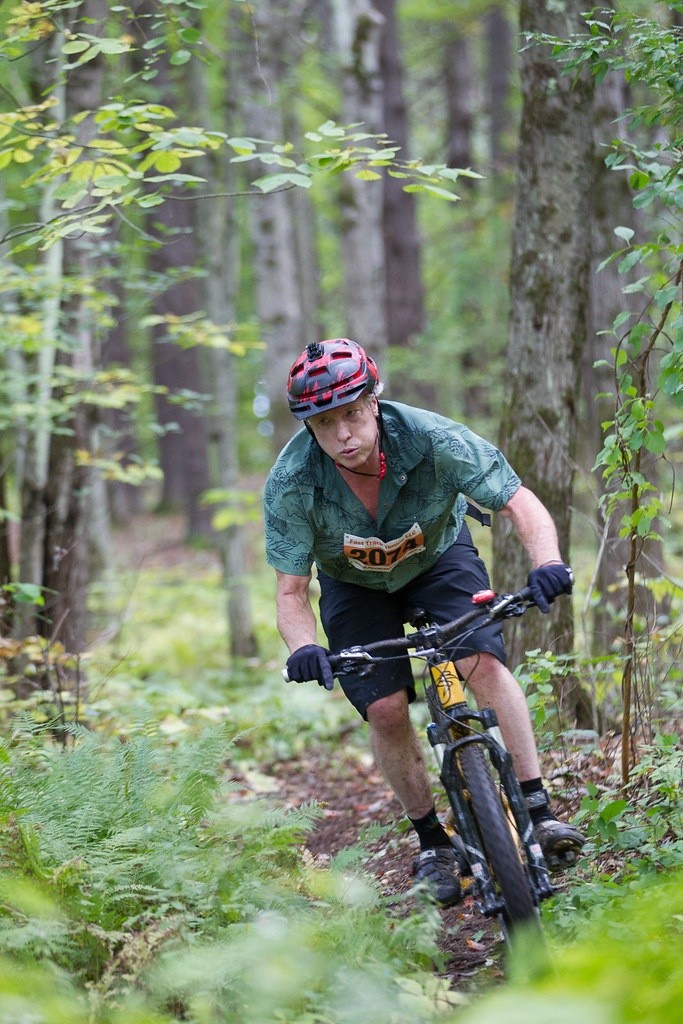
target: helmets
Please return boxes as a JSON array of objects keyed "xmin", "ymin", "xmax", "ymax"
[{"xmin": 286, "ymin": 338, "xmax": 378, "ymax": 420}]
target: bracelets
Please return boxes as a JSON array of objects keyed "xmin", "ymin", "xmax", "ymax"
[{"xmin": 538, "ymin": 559, "xmax": 562, "ymax": 567}]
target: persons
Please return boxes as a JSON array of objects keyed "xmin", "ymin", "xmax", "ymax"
[{"xmin": 263, "ymin": 338, "xmax": 584, "ymax": 905}]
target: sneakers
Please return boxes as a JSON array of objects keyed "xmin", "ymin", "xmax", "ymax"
[
  {"xmin": 411, "ymin": 840, "xmax": 460, "ymax": 908},
  {"xmin": 524, "ymin": 788, "xmax": 586, "ymax": 865}
]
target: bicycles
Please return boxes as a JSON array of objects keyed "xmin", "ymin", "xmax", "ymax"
[{"xmin": 280, "ymin": 566, "xmax": 579, "ymax": 979}]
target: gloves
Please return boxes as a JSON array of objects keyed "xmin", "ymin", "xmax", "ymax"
[
  {"xmin": 528, "ymin": 560, "xmax": 576, "ymax": 614},
  {"xmin": 286, "ymin": 644, "xmax": 339, "ymax": 690}
]
